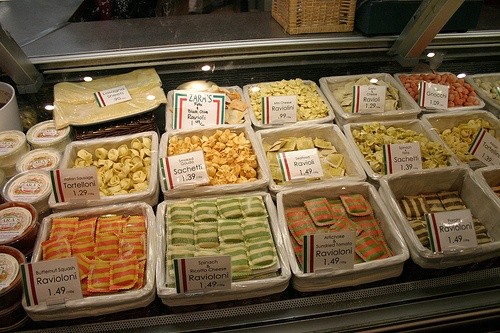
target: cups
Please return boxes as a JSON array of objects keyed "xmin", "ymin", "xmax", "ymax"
[{"xmin": 0, "ymin": 82, "xmax": 21, "ymax": 131}]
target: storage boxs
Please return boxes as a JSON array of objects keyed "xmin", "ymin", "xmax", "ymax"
[{"xmin": 21, "ymin": 73, "xmax": 500, "ymax": 327}]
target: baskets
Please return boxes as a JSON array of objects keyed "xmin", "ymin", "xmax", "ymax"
[
  {"xmin": 71, "ymin": 110, "xmax": 158, "ymax": 143},
  {"xmin": 271, "ymin": 0, "xmax": 356, "ymax": 34}
]
